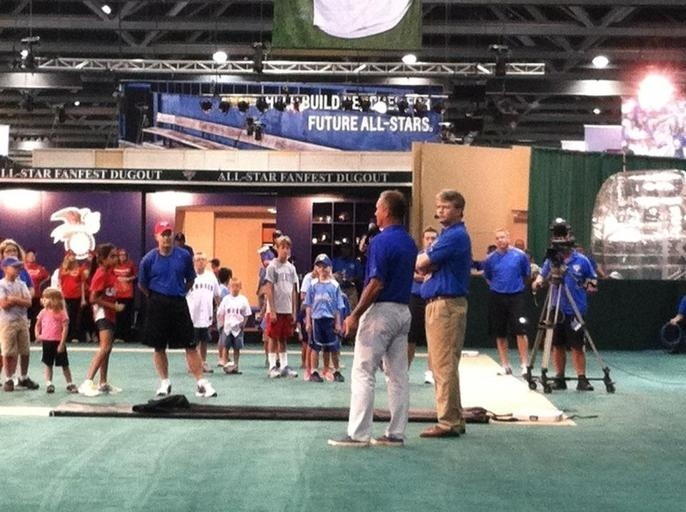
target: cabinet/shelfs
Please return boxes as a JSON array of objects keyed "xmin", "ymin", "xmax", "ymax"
[{"xmin": 278, "ymin": 188, "xmax": 383, "ymax": 288}]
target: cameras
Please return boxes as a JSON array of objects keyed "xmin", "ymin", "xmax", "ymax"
[{"xmin": 545, "ymin": 248, "xmax": 559, "ymax": 259}]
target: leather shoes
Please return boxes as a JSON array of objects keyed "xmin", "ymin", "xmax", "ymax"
[{"xmin": 419, "ymin": 425, "xmax": 465, "ymax": 440}]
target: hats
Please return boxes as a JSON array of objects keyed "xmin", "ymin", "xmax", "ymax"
[
  {"xmin": 153, "ymin": 220, "xmax": 173, "ymax": 234},
  {"xmin": 0, "ymin": 256, "xmax": 25, "ymax": 267},
  {"xmin": 314, "ymin": 253, "xmax": 332, "ymax": 265}
]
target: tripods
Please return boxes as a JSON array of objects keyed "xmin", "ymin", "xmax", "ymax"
[{"xmin": 522, "ymin": 260, "xmax": 615, "ymax": 393}]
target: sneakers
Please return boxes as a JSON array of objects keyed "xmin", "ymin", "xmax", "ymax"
[
  {"xmin": 4, "ymin": 377, "xmax": 40, "ymax": 392},
  {"xmin": 576, "ymin": 375, "xmax": 595, "ymax": 391},
  {"xmin": 46, "ymin": 383, "xmax": 56, "ymax": 393},
  {"xmin": 97, "ymin": 383, "xmax": 123, "ymax": 396},
  {"xmin": 201, "ymin": 359, "xmax": 345, "ymax": 382},
  {"xmin": 495, "ymin": 364, "xmax": 530, "ymax": 376},
  {"xmin": 369, "ymin": 434, "xmax": 406, "ymax": 448},
  {"xmin": 424, "ymin": 370, "xmax": 433, "ymax": 384},
  {"xmin": 77, "ymin": 379, "xmax": 100, "ymax": 397},
  {"xmin": 547, "ymin": 374, "xmax": 568, "ymax": 390},
  {"xmin": 66, "ymin": 383, "xmax": 79, "ymax": 394},
  {"xmin": 327, "ymin": 436, "xmax": 370, "ymax": 448},
  {"xmin": 155, "ymin": 378, "xmax": 172, "ymax": 397},
  {"xmin": 194, "ymin": 379, "xmax": 217, "ymax": 398}
]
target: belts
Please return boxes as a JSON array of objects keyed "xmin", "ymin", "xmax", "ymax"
[{"xmin": 425, "ymin": 295, "xmax": 455, "ymax": 305}]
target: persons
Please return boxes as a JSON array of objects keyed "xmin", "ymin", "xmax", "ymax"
[
  {"xmin": 670, "ymin": 295, "xmax": 686, "ymax": 325},
  {"xmin": 329, "ymin": 190, "xmax": 605, "ymax": 448},
  {"xmin": 0, "ymin": 221, "xmax": 218, "ymax": 398},
  {"xmin": 183, "ymin": 229, "xmax": 351, "ymax": 383}
]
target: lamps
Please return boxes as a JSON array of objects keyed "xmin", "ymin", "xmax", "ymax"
[
  {"xmin": 193, "ymin": 93, "xmax": 448, "ymax": 119},
  {"xmin": 244, "ymin": 122, "xmax": 266, "ymax": 142}
]
